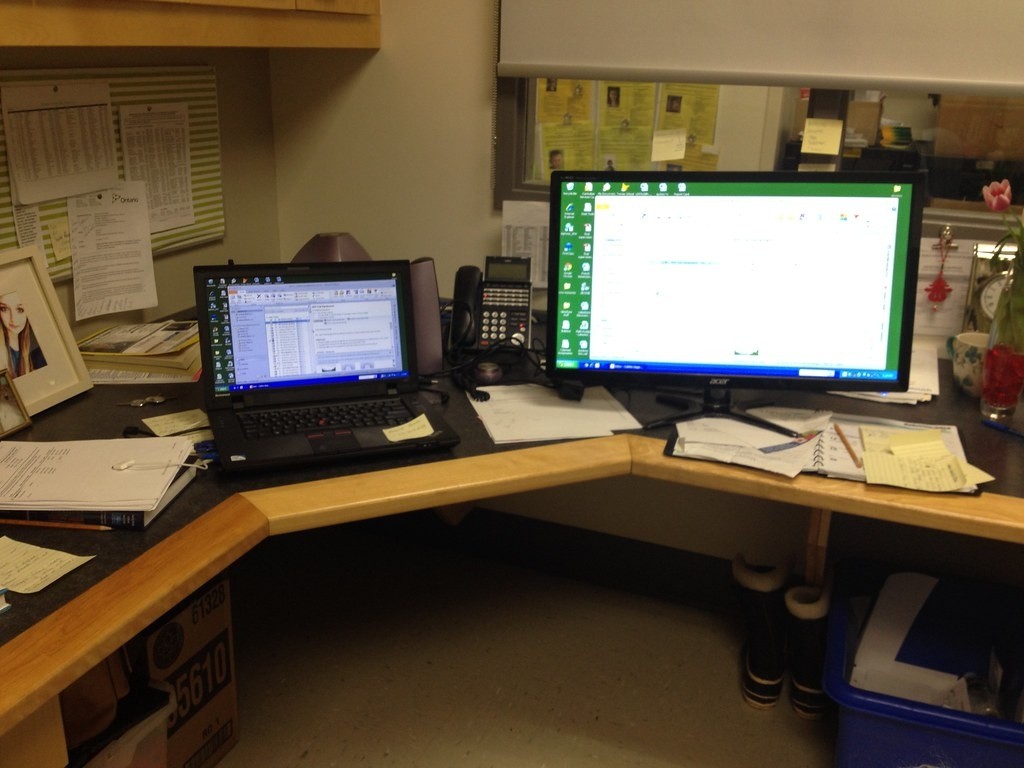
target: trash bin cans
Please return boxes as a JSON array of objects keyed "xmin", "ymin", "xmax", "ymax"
[{"xmin": 823, "ymin": 554, "xmax": 1024, "ymax": 768}]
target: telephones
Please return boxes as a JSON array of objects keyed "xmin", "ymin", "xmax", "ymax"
[{"xmin": 451, "ymin": 255, "xmax": 535, "ymax": 351}]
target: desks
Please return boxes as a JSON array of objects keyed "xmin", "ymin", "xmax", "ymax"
[{"xmin": 0, "ymin": 306, "xmax": 1023, "ymax": 768}]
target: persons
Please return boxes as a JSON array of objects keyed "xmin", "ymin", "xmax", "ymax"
[
  {"xmin": 0, "ymin": 284, "xmax": 48, "ymax": 380},
  {"xmin": 546, "ymin": 77, "xmax": 682, "ymax": 171}
]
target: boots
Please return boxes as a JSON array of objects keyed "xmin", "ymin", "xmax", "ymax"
[
  {"xmin": 733, "ymin": 554, "xmax": 783, "ymax": 710},
  {"xmin": 785, "ymin": 584, "xmax": 829, "ymax": 721}
]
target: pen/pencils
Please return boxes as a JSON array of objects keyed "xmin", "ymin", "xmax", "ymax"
[
  {"xmin": 982, "ymin": 419, "xmax": 1024, "ymax": 439},
  {"xmin": 0, "ymin": 518, "xmax": 113, "ymax": 530},
  {"xmin": 833, "ymin": 422, "xmax": 864, "ymax": 468}
]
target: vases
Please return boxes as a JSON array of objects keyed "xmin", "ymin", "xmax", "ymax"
[{"xmin": 978, "ymin": 259, "xmax": 1024, "ymax": 433}]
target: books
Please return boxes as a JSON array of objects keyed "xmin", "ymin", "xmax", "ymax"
[
  {"xmin": 76, "ymin": 320, "xmax": 203, "ymax": 386},
  {"xmin": 664, "ymin": 407, "xmax": 983, "ymax": 496}
]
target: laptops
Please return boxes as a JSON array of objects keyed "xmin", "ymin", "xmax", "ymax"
[{"xmin": 192, "ymin": 260, "xmax": 462, "ymax": 474}]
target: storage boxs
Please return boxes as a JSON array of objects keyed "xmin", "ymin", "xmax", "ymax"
[
  {"xmin": 820, "ymin": 557, "xmax": 1024, "ymax": 768},
  {"xmin": 845, "ymin": 92, "xmax": 887, "ymax": 147},
  {"xmin": 64, "ymin": 578, "xmax": 239, "ymax": 767}
]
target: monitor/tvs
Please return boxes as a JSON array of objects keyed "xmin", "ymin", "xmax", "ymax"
[{"xmin": 545, "ymin": 171, "xmax": 928, "ymax": 436}]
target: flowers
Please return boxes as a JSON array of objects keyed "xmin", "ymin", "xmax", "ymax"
[{"xmin": 983, "ymin": 179, "xmax": 1024, "ymax": 250}]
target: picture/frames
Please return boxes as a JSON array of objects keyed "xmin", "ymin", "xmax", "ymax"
[
  {"xmin": 0, "ymin": 244, "xmax": 93, "ymax": 416},
  {"xmin": 0, "ymin": 368, "xmax": 32, "ymax": 438}
]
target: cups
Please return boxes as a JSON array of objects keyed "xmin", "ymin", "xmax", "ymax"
[{"xmin": 946, "ymin": 331, "xmax": 991, "ymax": 396}]
